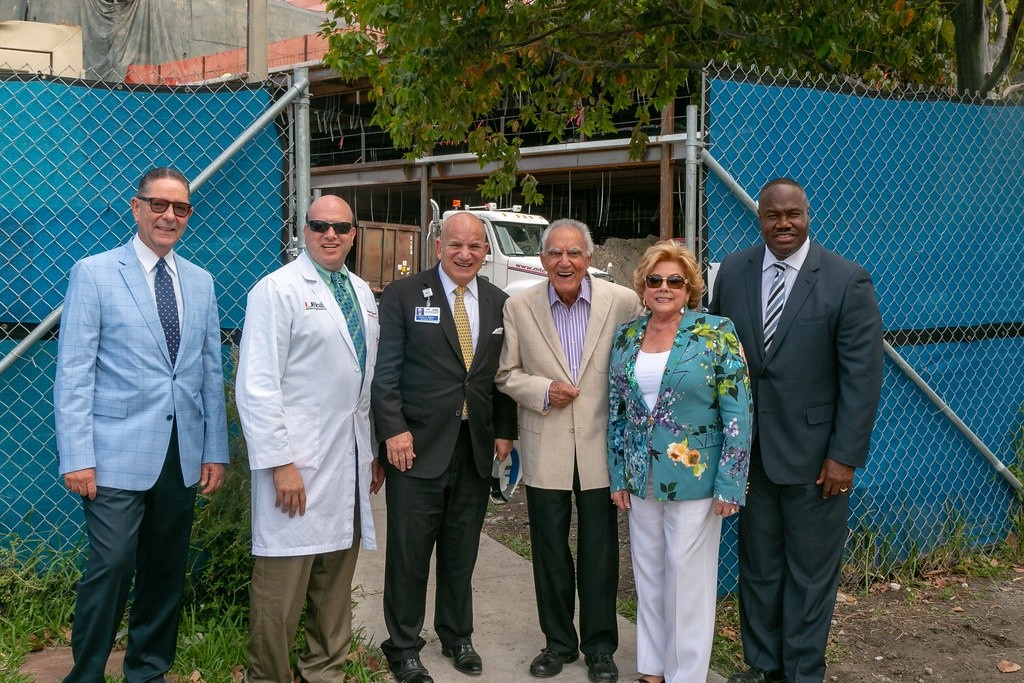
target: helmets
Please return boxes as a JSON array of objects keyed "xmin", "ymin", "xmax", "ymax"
[{"xmin": 490, "ymin": 437, "xmax": 523, "ymax": 504}]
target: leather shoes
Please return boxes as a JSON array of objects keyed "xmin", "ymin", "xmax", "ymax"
[
  {"xmin": 442, "ymin": 643, "xmax": 482, "ymax": 674},
  {"xmin": 389, "ymin": 657, "xmax": 434, "ymax": 683},
  {"xmin": 727, "ymin": 667, "xmax": 770, "ymax": 683},
  {"xmin": 584, "ymin": 654, "xmax": 619, "ymax": 683},
  {"xmin": 530, "ymin": 647, "xmax": 580, "ymax": 678}
]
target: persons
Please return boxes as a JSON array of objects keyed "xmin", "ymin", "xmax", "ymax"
[
  {"xmin": 494, "ymin": 219, "xmax": 644, "ymax": 683},
  {"xmin": 417, "ymin": 308, "xmax": 424, "ymax": 315},
  {"xmin": 52, "ymin": 167, "xmax": 229, "ymax": 683},
  {"xmin": 608, "ymin": 239, "xmax": 753, "ymax": 683},
  {"xmin": 708, "ymin": 177, "xmax": 884, "ymax": 683},
  {"xmin": 236, "ymin": 195, "xmax": 387, "ymax": 683},
  {"xmin": 370, "ymin": 212, "xmax": 519, "ymax": 683}
]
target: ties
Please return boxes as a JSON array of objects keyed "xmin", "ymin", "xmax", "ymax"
[
  {"xmin": 452, "ymin": 288, "xmax": 475, "ymax": 420},
  {"xmin": 330, "ymin": 272, "xmax": 367, "ymax": 395},
  {"xmin": 763, "ymin": 262, "xmax": 789, "ymax": 354},
  {"xmin": 154, "ymin": 258, "xmax": 181, "ymax": 368}
]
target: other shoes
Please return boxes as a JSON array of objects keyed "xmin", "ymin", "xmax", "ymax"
[{"xmin": 632, "ymin": 676, "xmax": 665, "ymax": 683}]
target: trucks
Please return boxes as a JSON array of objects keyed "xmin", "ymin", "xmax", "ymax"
[{"xmin": 424, "ymin": 198, "xmax": 615, "ymax": 296}]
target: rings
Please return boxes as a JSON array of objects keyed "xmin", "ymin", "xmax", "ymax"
[
  {"xmin": 730, "ymin": 509, "xmax": 735, "ymax": 514},
  {"xmin": 840, "ymin": 487, "xmax": 848, "ymax": 493},
  {"xmin": 613, "ymin": 502, "xmax": 615, "ymax": 504}
]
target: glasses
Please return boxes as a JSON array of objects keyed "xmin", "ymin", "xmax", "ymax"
[
  {"xmin": 646, "ymin": 274, "xmax": 688, "ymax": 289},
  {"xmin": 307, "ymin": 220, "xmax": 353, "ymax": 234},
  {"xmin": 138, "ymin": 196, "xmax": 193, "ymax": 217}
]
